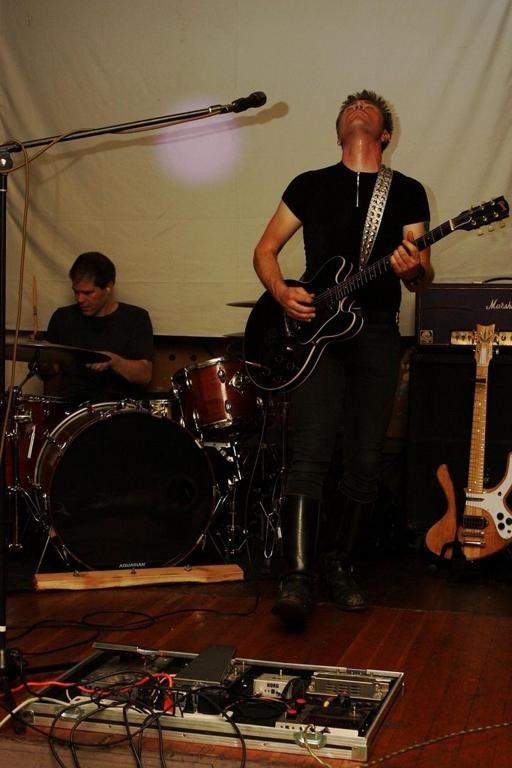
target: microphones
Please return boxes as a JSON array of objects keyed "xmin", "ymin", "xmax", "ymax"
[{"xmin": 230, "ymin": 90, "xmax": 269, "ymax": 111}]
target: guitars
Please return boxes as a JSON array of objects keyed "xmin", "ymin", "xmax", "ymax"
[
  {"xmin": 426, "ymin": 326, "xmax": 512, "ymax": 564},
  {"xmin": 241, "ymin": 196, "xmax": 510, "ymax": 394}
]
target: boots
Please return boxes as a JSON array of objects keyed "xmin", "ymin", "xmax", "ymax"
[
  {"xmin": 332, "ymin": 501, "xmax": 372, "ymax": 611},
  {"xmin": 271, "ymin": 494, "xmax": 320, "ymax": 622}
]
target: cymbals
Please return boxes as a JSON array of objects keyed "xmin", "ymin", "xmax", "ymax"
[
  {"xmin": 224, "ymin": 299, "xmax": 260, "ymax": 312},
  {"xmin": 4, "ymin": 336, "xmax": 112, "ymax": 370}
]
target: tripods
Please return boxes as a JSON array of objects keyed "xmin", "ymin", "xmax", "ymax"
[{"xmin": 0, "ymin": 104, "xmax": 230, "ymax": 736}]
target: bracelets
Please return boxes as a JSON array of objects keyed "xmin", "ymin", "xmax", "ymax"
[{"xmin": 402, "ymin": 267, "xmax": 427, "ymax": 287}]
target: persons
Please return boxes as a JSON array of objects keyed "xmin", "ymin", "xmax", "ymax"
[
  {"xmin": 253, "ymin": 87, "xmax": 433, "ymax": 620},
  {"xmin": 35, "ymin": 252, "xmax": 158, "ymax": 401}
]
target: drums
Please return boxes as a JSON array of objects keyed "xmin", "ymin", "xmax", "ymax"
[
  {"xmin": 38, "ymin": 398, "xmax": 217, "ymax": 571},
  {"xmin": 169, "ymin": 358, "xmax": 244, "ymax": 443},
  {"xmin": 0, "ymin": 391, "xmax": 69, "ymax": 489}
]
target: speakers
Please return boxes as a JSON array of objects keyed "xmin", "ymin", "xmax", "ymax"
[{"xmin": 405, "ymin": 349, "xmax": 511, "ymax": 539}]
